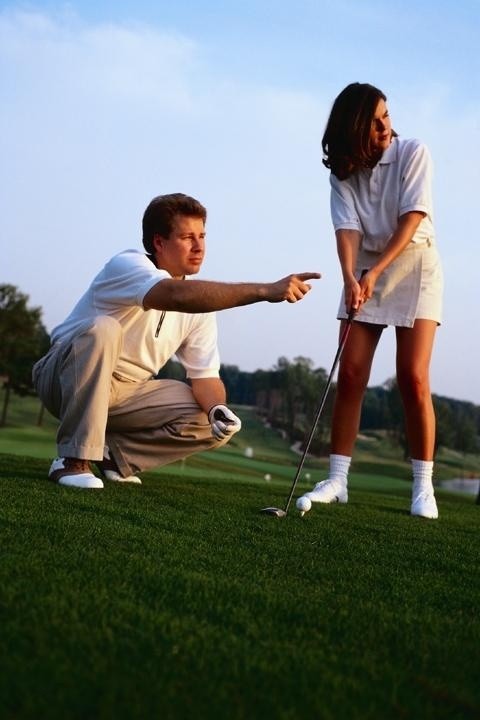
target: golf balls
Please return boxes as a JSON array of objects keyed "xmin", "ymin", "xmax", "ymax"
[{"xmin": 296, "ymin": 497, "xmax": 311, "ymax": 511}]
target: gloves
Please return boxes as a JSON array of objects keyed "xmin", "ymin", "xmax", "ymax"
[{"xmin": 208, "ymin": 404, "xmax": 241, "ymax": 442}]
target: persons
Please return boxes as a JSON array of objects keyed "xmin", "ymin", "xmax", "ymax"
[
  {"xmin": 302, "ymin": 81, "xmax": 441, "ymax": 519},
  {"xmin": 32, "ymin": 192, "xmax": 320, "ymax": 489}
]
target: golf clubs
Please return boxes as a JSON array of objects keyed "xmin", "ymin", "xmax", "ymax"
[{"xmin": 261, "ymin": 269, "xmax": 369, "ymax": 519}]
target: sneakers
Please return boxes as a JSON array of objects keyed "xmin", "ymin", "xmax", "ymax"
[
  {"xmin": 303, "ymin": 479, "xmax": 348, "ymax": 503},
  {"xmin": 411, "ymin": 492, "xmax": 438, "ymax": 519},
  {"xmin": 47, "ymin": 445, "xmax": 142, "ymax": 489}
]
